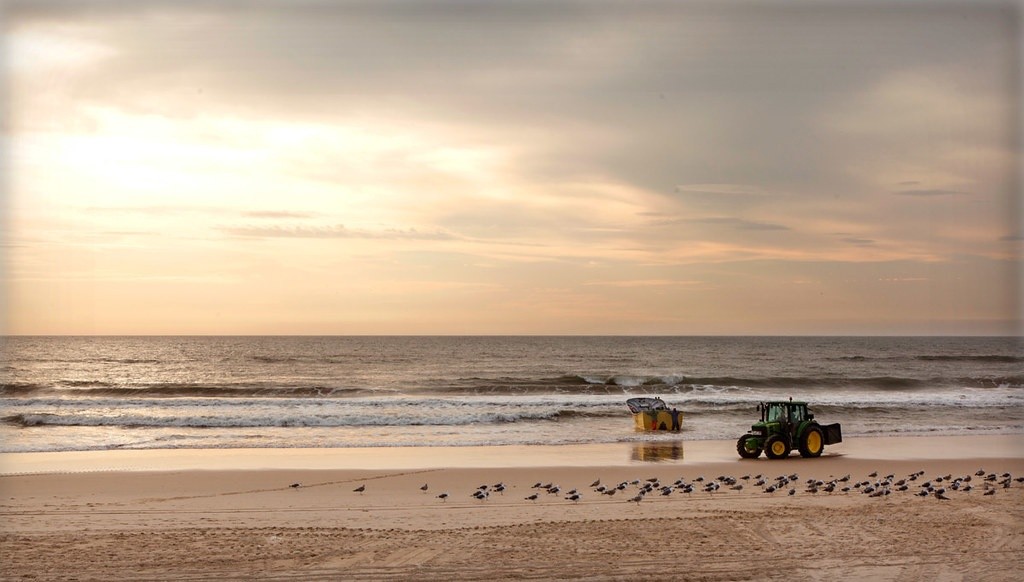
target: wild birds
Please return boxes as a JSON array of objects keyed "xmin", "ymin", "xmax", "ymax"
[{"xmin": 289, "ymin": 466, "xmax": 1024, "ymax": 507}]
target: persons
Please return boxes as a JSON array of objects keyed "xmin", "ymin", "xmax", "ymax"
[
  {"xmin": 667, "ymin": 408, "xmax": 681, "ymax": 432},
  {"xmin": 646, "ymin": 409, "xmax": 659, "ymax": 431}
]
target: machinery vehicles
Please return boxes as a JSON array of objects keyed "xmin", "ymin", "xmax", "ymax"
[{"xmin": 735, "ymin": 396, "xmax": 844, "ymax": 458}]
